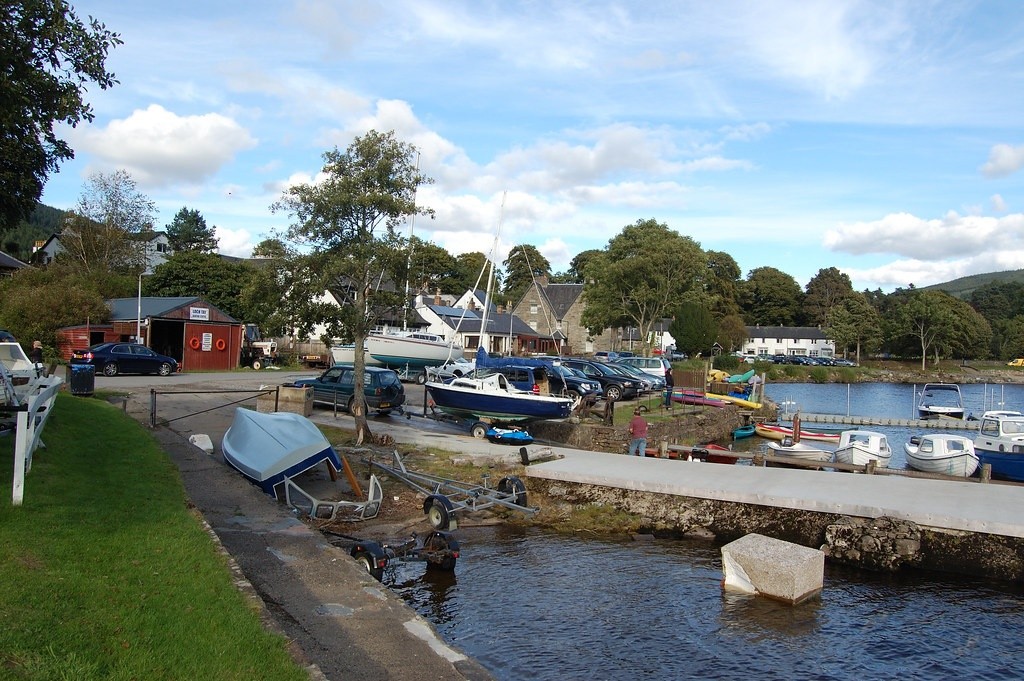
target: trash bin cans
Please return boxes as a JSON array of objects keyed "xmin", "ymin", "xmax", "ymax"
[{"xmin": 66, "ymin": 364, "xmax": 95, "ymax": 397}]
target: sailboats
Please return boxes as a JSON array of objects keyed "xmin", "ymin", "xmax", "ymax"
[
  {"xmin": 364, "ymin": 149, "xmax": 464, "ymax": 367},
  {"xmin": 431, "ymin": 184, "xmax": 511, "ymax": 379}
]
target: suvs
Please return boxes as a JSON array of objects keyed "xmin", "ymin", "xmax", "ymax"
[{"xmin": 295, "ymin": 365, "xmax": 405, "ymax": 418}]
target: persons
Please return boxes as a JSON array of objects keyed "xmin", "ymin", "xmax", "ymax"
[
  {"xmin": 750, "ymin": 419, "xmax": 756, "ymax": 425},
  {"xmin": 628, "ymin": 411, "xmax": 648, "ymax": 457},
  {"xmin": 27, "ymin": 341, "xmax": 43, "ymax": 369},
  {"xmin": 666, "ymin": 368, "xmax": 674, "ymax": 410},
  {"xmin": 533, "ymin": 383, "xmax": 540, "ymax": 396}
]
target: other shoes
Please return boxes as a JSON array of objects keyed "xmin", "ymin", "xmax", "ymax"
[{"xmin": 666, "ymin": 404, "xmax": 671, "ymax": 406}]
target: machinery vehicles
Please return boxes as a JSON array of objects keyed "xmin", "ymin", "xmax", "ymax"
[{"xmin": 240, "ymin": 323, "xmax": 280, "ymax": 370}]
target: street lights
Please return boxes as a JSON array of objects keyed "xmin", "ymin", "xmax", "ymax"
[{"xmin": 137, "ymin": 272, "xmax": 154, "ymax": 343}]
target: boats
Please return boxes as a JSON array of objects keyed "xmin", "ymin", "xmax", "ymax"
[
  {"xmin": 754, "ymin": 424, "xmax": 840, "ymax": 442},
  {"xmin": 974, "ymin": 411, "xmax": 1024, "ymax": 483},
  {"xmin": 222, "ymin": 407, "xmax": 344, "ymax": 498},
  {"xmin": 917, "ymin": 381, "xmax": 964, "ymax": 420},
  {"xmin": 330, "ymin": 339, "xmax": 382, "ymax": 368},
  {"xmin": 632, "ymin": 444, "xmax": 738, "ymax": 464},
  {"xmin": 425, "ymin": 372, "xmax": 573, "ymax": 423},
  {"xmin": 768, "ymin": 439, "xmax": 832, "ymax": 469},
  {"xmin": 834, "ymin": 430, "xmax": 893, "ymax": 474},
  {"xmin": 733, "ymin": 425, "xmax": 755, "ymax": 438},
  {"xmin": 486, "ymin": 427, "xmax": 534, "ymax": 446},
  {"xmin": 707, "ymin": 369, "xmax": 762, "ymax": 385},
  {"xmin": 663, "ymin": 389, "xmax": 763, "ymax": 409},
  {"xmin": 903, "ymin": 433, "xmax": 979, "ymax": 478}
]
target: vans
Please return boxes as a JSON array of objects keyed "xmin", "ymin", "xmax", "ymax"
[
  {"xmin": 1006, "ymin": 359, "xmax": 1024, "ymax": 366},
  {"xmin": 613, "ymin": 357, "xmax": 671, "ymax": 383}
]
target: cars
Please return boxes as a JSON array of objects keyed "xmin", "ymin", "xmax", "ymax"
[
  {"xmin": 532, "ymin": 358, "xmax": 602, "ymax": 404},
  {"xmin": 834, "ymin": 358, "xmax": 857, "ymax": 367},
  {"xmin": 601, "ymin": 363, "xmax": 665, "ymax": 396},
  {"xmin": 594, "ymin": 350, "xmax": 617, "ymax": 362},
  {"xmin": 561, "ymin": 358, "xmax": 643, "ymax": 402},
  {"xmin": 611, "ymin": 351, "xmax": 633, "ymax": 361},
  {"xmin": 755, "ymin": 354, "xmax": 837, "ymax": 367},
  {"xmin": 738, "ymin": 355, "xmax": 764, "ymax": 363},
  {"xmin": 672, "ymin": 351, "xmax": 684, "ymax": 359},
  {"xmin": 0, "ymin": 342, "xmax": 45, "ymax": 410},
  {"xmin": 442, "ymin": 365, "xmax": 551, "ymax": 397},
  {"xmin": 70, "ymin": 342, "xmax": 179, "ymax": 377}
]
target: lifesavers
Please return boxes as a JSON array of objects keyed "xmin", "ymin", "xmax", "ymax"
[
  {"xmin": 190, "ymin": 338, "xmax": 200, "ymax": 350},
  {"xmin": 215, "ymin": 338, "xmax": 226, "ymax": 351}
]
